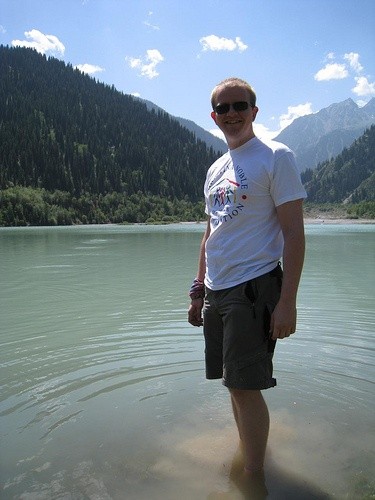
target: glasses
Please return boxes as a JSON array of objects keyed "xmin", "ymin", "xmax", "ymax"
[{"xmin": 214, "ymin": 101, "xmax": 253, "ymax": 114}]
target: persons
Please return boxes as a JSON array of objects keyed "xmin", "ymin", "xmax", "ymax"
[{"xmin": 187, "ymin": 76, "xmax": 305, "ymax": 473}]
